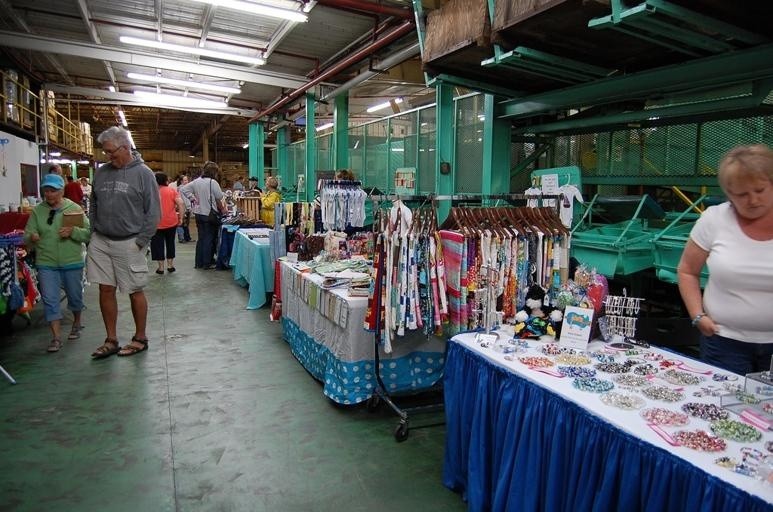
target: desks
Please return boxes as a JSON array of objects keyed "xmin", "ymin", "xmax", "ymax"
[
  {"xmin": 236, "ymin": 229, "xmax": 273, "ymax": 301},
  {"xmin": 448, "ymin": 333, "xmax": 773, "ymax": 512},
  {"xmin": 279, "ymin": 256, "xmax": 454, "ymax": 409}
]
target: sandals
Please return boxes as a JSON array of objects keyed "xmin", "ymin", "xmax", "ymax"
[
  {"xmin": 116, "ymin": 338, "xmax": 149, "ymax": 358},
  {"xmin": 66, "ymin": 323, "xmax": 83, "ymax": 341},
  {"xmin": 47, "ymin": 339, "xmax": 65, "ymax": 355},
  {"xmin": 90, "ymin": 337, "xmax": 120, "ymax": 362}
]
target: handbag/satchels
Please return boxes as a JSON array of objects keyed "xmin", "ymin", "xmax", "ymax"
[{"xmin": 208, "ymin": 178, "xmax": 220, "ymax": 225}]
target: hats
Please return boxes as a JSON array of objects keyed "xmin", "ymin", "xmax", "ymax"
[
  {"xmin": 39, "ymin": 173, "xmax": 67, "ymax": 192},
  {"xmin": 248, "ymin": 175, "xmax": 259, "ymax": 182}
]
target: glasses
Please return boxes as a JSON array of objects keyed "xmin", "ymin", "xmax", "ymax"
[
  {"xmin": 99, "ymin": 145, "xmax": 126, "ymax": 160},
  {"xmin": 47, "ymin": 208, "xmax": 57, "ymax": 226}
]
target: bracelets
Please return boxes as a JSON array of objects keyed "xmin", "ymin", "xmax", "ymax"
[{"xmin": 691, "ymin": 315, "xmax": 705, "ymax": 324}]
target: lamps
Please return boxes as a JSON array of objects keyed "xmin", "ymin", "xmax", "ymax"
[
  {"xmin": 195, "ymin": 0, "xmax": 309, "ymax": 24},
  {"xmin": 124, "ymin": 72, "xmax": 242, "ymax": 95},
  {"xmin": 134, "ymin": 91, "xmax": 228, "ymax": 110},
  {"xmin": 119, "ymin": 35, "xmax": 267, "ymax": 67}
]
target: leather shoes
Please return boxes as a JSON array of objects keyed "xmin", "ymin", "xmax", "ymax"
[
  {"xmin": 156, "ymin": 267, "xmax": 165, "ymax": 275},
  {"xmin": 168, "ymin": 266, "xmax": 177, "ymax": 273}
]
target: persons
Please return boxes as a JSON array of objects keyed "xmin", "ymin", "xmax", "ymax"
[
  {"xmin": 177, "ymin": 174, "xmax": 196, "ymax": 242},
  {"xmin": 151, "ymin": 172, "xmax": 185, "ymax": 274},
  {"xmin": 260, "ymin": 177, "xmax": 281, "ymax": 224},
  {"xmin": 80, "ymin": 177, "xmax": 92, "ymax": 216},
  {"xmin": 333, "ymin": 168, "xmax": 354, "ymax": 180},
  {"xmin": 85, "ymin": 126, "xmax": 161, "ymax": 357},
  {"xmin": 65, "ymin": 176, "xmax": 83, "ymax": 205},
  {"xmin": 249, "ymin": 176, "xmax": 262, "ymax": 192},
  {"xmin": 233, "ymin": 177, "xmax": 245, "ymax": 190},
  {"xmin": 677, "ymin": 144, "xmax": 773, "ymax": 380},
  {"xmin": 48, "ymin": 164, "xmax": 63, "ymax": 176},
  {"xmin": 180, "ymin": 162, "xmax": 228, "ymax": 269},
  {"xmin": 23, "ymin": 174, "xmax": 90, "ymax": 351}
]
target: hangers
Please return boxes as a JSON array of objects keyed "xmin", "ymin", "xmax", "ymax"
[
  {"xmin": 317, "ymin": 179, "xmax": 361, "ymax": 190},
  {"xmin": 369, "ymin": 193, "xmax": 573, "ymax": 242}
]
